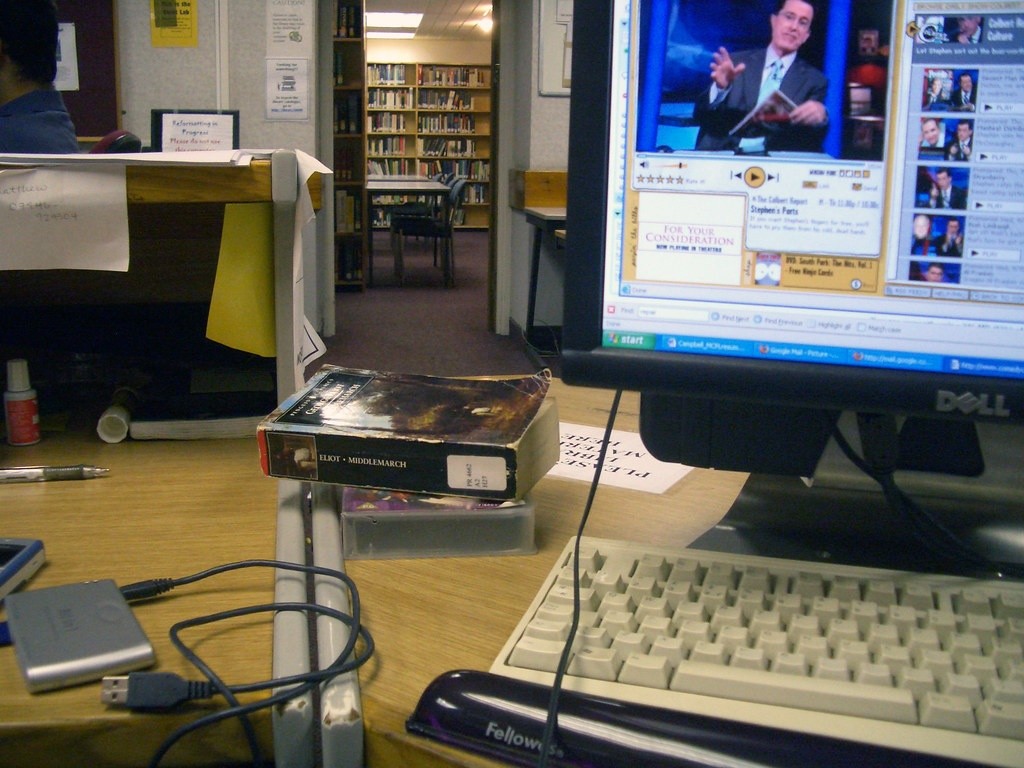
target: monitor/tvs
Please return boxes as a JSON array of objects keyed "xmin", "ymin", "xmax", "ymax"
[{"xmin": 555, "ymin": 0, "xmax": 1024, "ymax": 584}]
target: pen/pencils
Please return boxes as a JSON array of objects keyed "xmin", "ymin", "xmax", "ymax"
[{"xmin": 0, "ymin": 462, "xmax": 112, "ymax": 485}]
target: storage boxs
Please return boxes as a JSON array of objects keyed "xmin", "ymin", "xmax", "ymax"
[{"xmin": 340, "ymin": 485, "xmax": 536, "ymax": 559}]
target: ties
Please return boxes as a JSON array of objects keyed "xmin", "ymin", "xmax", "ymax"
[
  {"xmin": 758, "ymin": 59, "xmax": 783, "ymax": 104},
  {"xmin": 944, "ymin": 190, "xmax": 948, "ymax": 200},
  {"xmin": 964, "ymin": 93, "xmax": 968, "ymax": 104},
  {"xmin": 962, "ymin": 142, "xmax": 965, "ymax": 149}
]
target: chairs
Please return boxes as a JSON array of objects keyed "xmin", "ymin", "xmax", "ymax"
[{"xmin": 387, "ymin": 174, "xmax": 468, "ymax": 282}]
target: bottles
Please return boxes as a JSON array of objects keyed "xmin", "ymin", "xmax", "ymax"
[{"xmin": 4, "ymin": 358, "xmax": 40, "ymax": 446}]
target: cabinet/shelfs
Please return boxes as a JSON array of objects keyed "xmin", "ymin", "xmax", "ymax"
[
  {"xmin": 366, "ymin": 62, "xmax": 491, "ymax": 231},
  {"xmin": 334, "ymin": 0, "xmax": 372, "ymax": 292}
]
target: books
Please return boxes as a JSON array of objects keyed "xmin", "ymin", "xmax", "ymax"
[
  {"xmin": 368, "ymin": 88, "xmax": 413, "ymax": 109},
  {"xmin": 367, "ymin": 64, "xmax": 406, "ymax": 85},
  {"xmin": 418, "ymin": 65, "xmax": 484, "ymax": 87},
  {"xmin": 254, "ymin": 362, "xmax": 560, "ymax": 501},
  {"xmin": 418, "ymin": 112, "xmax": 475, "ymax": 133},
  {"xmin": 129, "ymin": 391, "xmax": 276, "ymax": 440},
  {"xmin": 418, "ymin": 89, "xmax": 474, "ymax": 110},
  {"xmin": 418, "ymin": 137, "xmax": 476, "ymax": 158},
  {"xmin": 332, "ymin": 0, "xmax": 362, "ymax": 282},
  {"xmin": 369, "ymin": 136, "xmax": 406, "ymax": 155},
  {"xmin": 367, "ymin": 112, "xmax": 406, "ymax": 133},
  {"xmin": 367, "ymin": 159, "xmax": 408, "ymax": 227},
  {"xmin": 420, "ymin": 160, "xmax": 490, "ymax": 226},
  {"xmin": 728, "ymin": 89, "xmax": 804, "ymax": 146}
]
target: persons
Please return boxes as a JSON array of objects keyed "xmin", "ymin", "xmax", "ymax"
[
  {"xmin": 926, "ymin": 262, "xmax": 944, "ymax": 282},
  {"xmin": 925, "ymin": 168, "xmax": 967, "ymax": 209},
  {"xmin": 933, "ymin": 219, "xmax": 963, "ymax": 257},
  {"xmin": 691, "ymin": 0, "xmax": 830, "ymax": 154},
  {"xmin": 947, "ymin": 120, "xmax": 973, "ymax": 161},
  {"xmin": 0, "ymin": 0, "xmax": 79, "ymax": 153},
  {"xmin": 926, "ymin": 73, "xmax": 976, "ymax": 112},
  {"xmin": 918, "ymin": 119, "xmax": 945, "ymax": 148},
  {"xmin": 948, "ymin": 16, "xmax": 983, "ymax": 44}
]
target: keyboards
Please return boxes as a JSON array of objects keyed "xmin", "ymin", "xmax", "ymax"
[{"xmin": 488, "ymin": 536, "xmax": 1024, "ymax": 768}]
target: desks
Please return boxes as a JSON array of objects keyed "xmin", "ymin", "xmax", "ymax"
[
  {"xmin": 525, "ymin": 205, "xmax": 567, "ymax": 375},
  {"xmin": 0, "ymin": 376, "xmax": 749, "ymax": 767},
  {"xmin": 366, "ymin": 181, "xmax": 452, "ymax": 289},
  {"xmin": 367, "ymin": 175, "xmax": 431, "ymax": 182},
  {"xmin": 0, "ymin": 160, "xmax": 324, "ymax": 406}
]
